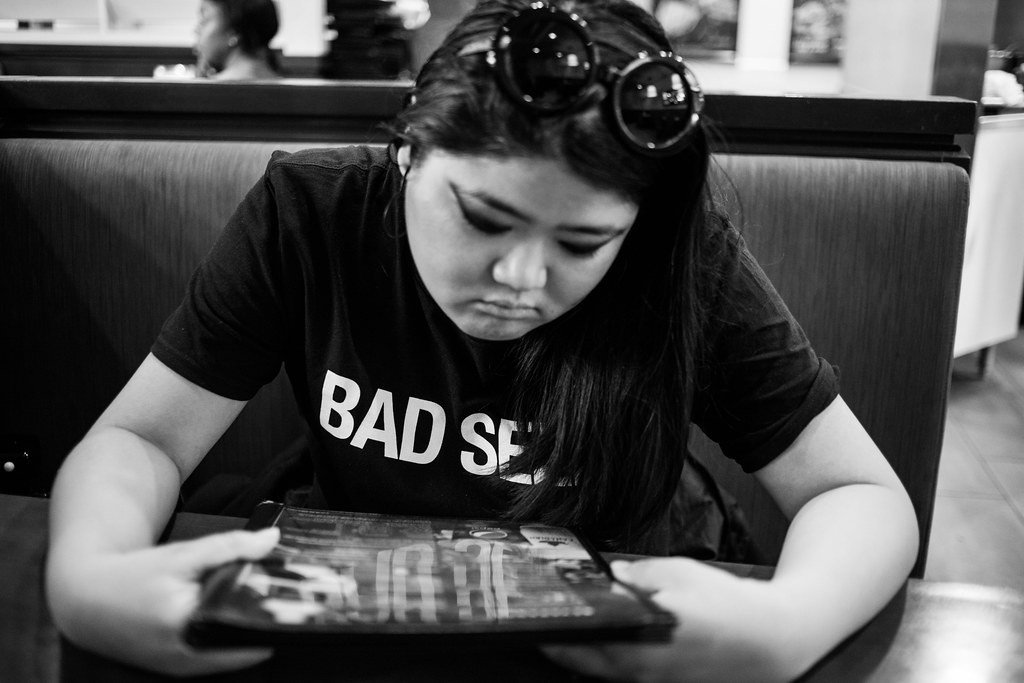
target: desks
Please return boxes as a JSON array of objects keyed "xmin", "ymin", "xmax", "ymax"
[{"xmin": 0, "ymin": 497, "xmax": 1024, "ymax": 683}]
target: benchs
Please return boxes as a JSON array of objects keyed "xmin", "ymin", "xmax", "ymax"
[{"xmin": 0, "ymin": 43, "xmax": 994, "ymax": 572}]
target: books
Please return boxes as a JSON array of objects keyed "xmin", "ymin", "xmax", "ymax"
[{"xmin": 179, "ymin": 500, "xmax": 682, "ymax": 657}]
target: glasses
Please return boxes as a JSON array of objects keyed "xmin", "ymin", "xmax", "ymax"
[{"xmin": 455, "ymin": 1, "xmax": 704, "ymax": 151}]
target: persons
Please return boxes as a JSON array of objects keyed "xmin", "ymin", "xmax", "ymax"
[
  {"xmin": 47, "ymin": 0, "xmax": 919, "ymax": 683},
  {"xmin": 191, "ymin": 0, "xmax": 295, "ymax": 78}
]
